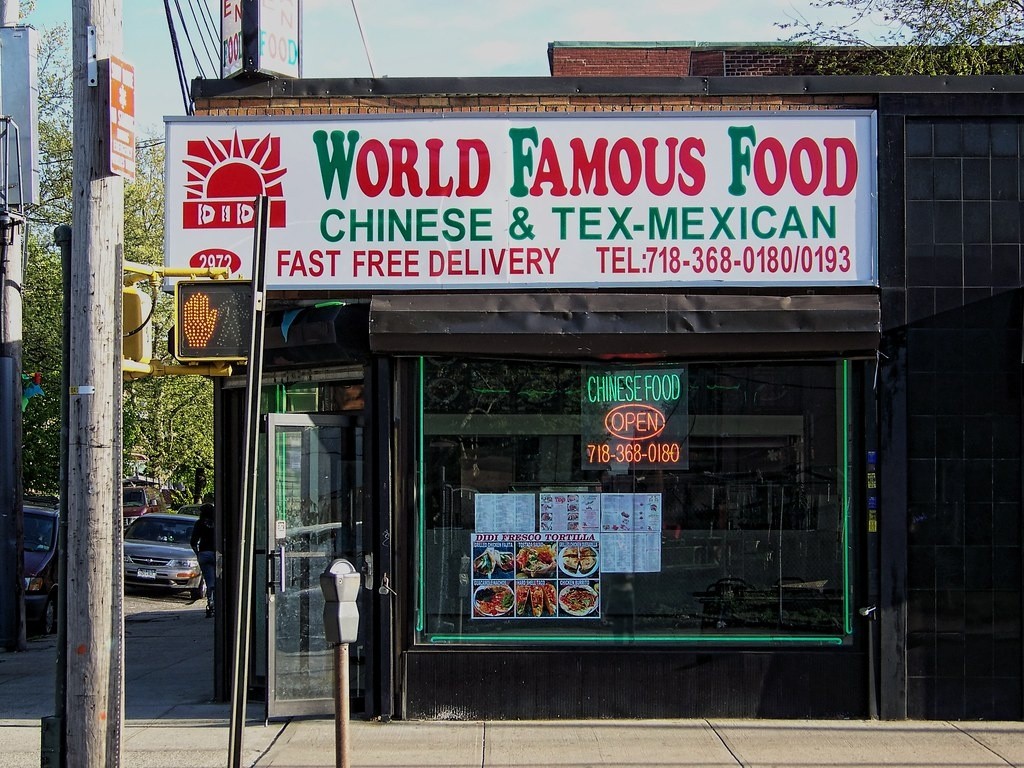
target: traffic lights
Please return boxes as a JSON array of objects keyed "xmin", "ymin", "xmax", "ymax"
[{"xmin": 174, "ymin": 280, "xmax": 250, "ymax": 362}]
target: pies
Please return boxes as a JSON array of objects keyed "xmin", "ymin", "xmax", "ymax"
[
  {"xmin": 559, "ymin": 584, "xmax": 599, "ymax": 616},
  {"xmin": 563, "ymin": 546, "xmax": 597, "ymax": 571},
  {"xmin": 474, "ymin": 546, "xmax": 513, "ymax": 576},
  {"xmin": 516, "ymin": 583, "xmax": 557, "ymax": 617}
]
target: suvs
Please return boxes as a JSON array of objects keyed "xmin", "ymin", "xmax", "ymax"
[{"xmin": 122, "ymin": 485, "xmax": 162, "ymax": 534}]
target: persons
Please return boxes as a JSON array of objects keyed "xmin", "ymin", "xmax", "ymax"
[
  {"xmin": 190, "ymin": 503, "xmax": 216, "ymax": 605},
  {"xmin": 610, "ymin": 533, "xmax": 636, "ymax": 646}
]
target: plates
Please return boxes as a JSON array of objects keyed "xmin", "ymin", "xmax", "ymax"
[
  {"xmin": 473, "ymin": 584, "xmax": 514, "ymax": 616},
  {"xmin": 558, "ymin": 546, "xmax": 598, "ymax": 577},
  {"xmin": 558, "ymin": 585, "xmax": 598, "ymax": 616}
]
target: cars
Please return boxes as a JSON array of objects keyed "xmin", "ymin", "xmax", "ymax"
[
  {"xmin": 22, "ymin": 499, "xmax": 60, "ymax": 634},
  {"xmin": 124, "ymin": 513, "xmax": 208, "ymax": 599}
]
